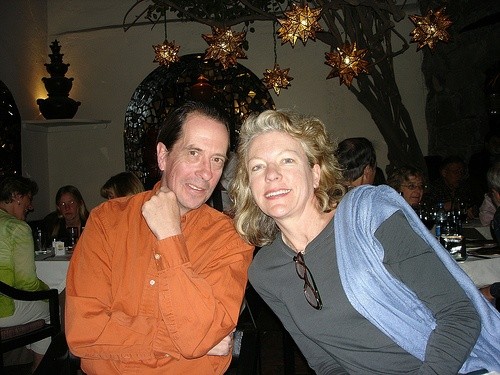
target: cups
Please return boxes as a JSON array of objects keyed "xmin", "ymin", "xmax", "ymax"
[{"xmin": 54, "ymin": 241, "xmax": 66, "ymax": 256}]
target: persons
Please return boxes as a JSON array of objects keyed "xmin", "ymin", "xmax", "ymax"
[
  {"xmin": 428, "ymin": 155, "xmax": 478, "ymax": 214},
  {"xmin": 101, "ymin": 171, "xmax": 145, "ymax": 201},
  {"xmin": 227, "ymin": 109, "xmax": 500, "ymax": 375},
  {"xmin": 0, "ymin": 176, "xmax": 51, "ymax": 375},
  {"xmin": 45, "ymin": 185, "xmax": 90, "ymax": 249},
  {"xmin": 64, "ymin": 100, "xmax": 254, "ymax": 375},
  {"xmin": 334, "ymin": 137, "xmax": 376, "ymax": 186},
  {"xmin": 389, "ymin": 164, "xmax": 424, "ymax": 207},
  {"xmin": 479, "ymin": 162, "xmax": 500, "ymax": 312}
]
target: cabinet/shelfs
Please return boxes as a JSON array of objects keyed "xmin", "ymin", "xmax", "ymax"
[{"xmin": 22, "ymin": 120, "xmax": 108, "ymax": 215}]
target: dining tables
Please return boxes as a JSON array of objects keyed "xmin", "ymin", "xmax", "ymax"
[
  {"xmin": 457, "ymin": 246, "xmax": 500, "ymax": 290},
  {"xmin": 35, "ymin": 246, "xmax": 74, "ymax": 328}
]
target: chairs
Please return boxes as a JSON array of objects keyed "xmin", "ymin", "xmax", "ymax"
[{"xmin": 0, "ymin": 281, "xmax": 61, "ymax": 375}]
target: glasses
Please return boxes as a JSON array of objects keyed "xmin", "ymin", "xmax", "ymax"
[
  {"xmin": 57, "ymin": 200, "xmax": 78, "ymax": 207},
  {"xmin": 292, "ymin": 251, "xmax": 324, "ymax": 311},
  {"xmin": 399, "ymin": 184, "xmax": 425, "ymax": 190}
]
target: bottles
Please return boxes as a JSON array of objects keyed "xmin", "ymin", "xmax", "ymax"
[{"xmin": 417, "ymin": 199, "xmax": 468, "ymax": 243}]
